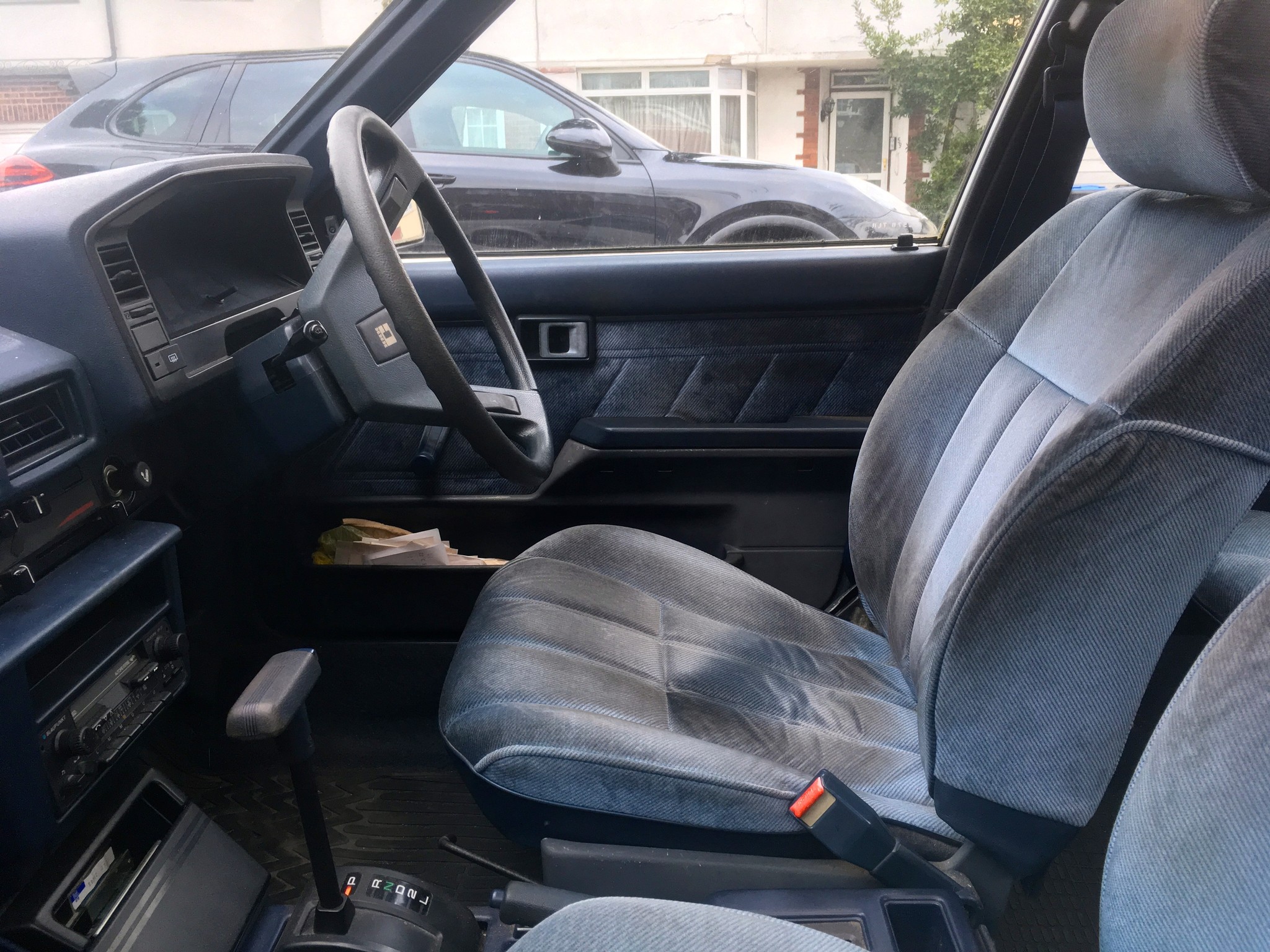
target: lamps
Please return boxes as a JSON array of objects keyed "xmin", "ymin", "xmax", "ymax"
[{"xmin": 820, "ymin": 95, "xmax": 836, "ymax": 121}]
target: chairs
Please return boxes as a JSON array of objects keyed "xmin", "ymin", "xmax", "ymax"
[
  {"xmin": 506, "ymin": 576, "xmax": 1270, "ymax": 952},
  {"xmin": 438, "ymin": 1, "xmax": 1270, "ymax": 898}
]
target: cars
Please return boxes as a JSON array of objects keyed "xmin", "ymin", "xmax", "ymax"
[{"xmin": 0, "ymin": 49, "xmax": 939, "ymax": 261}]
